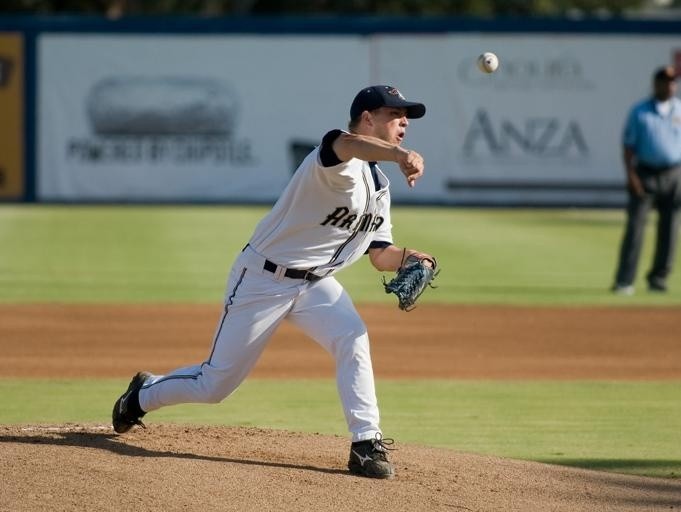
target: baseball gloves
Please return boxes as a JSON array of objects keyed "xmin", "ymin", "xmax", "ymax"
[{"xmin": 382, "ymin": 248, "xmax": 442, "ymax": 312}]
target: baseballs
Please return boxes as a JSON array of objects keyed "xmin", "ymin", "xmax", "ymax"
[{"xmin": 479, "ymin": 52, "xmax": 498, "ymax": 74}]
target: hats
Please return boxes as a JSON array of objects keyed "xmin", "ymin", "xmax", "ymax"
[
  {"xmin": 349, "ymin": 85, "xmax": 425, "ymax": 119},
  {"xmin": 653, "ymin": 65, "xmax": 679, "ymax": 85}
]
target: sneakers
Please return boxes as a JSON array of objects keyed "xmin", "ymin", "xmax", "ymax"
[
  {"xmin": 347, "ymin": 432, "xmax": 398, "ymax": 479},
  {"xmin": 611, "ymin": 283, "xmax": 633, "ymax": 295},
  {"xmin": 645, "ymin": 273, "xmax": 667, "ymax": 291},
  {"xmin": 112, "ymin": 370, "xmax": 152, "ymax": 434}
]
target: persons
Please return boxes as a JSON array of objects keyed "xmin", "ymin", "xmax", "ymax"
[
  {"xmin": 109, "ymin": 86, "xmax": 437, "ymax": 481},
  {"xmin": 610, "ymin": 64, "xmax": 681, "ymax": 297}
]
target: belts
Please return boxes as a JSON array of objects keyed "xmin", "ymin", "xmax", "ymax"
[{"xmin": 241, "ymin": 243, "xmax": 318, "ymax": 281}]
